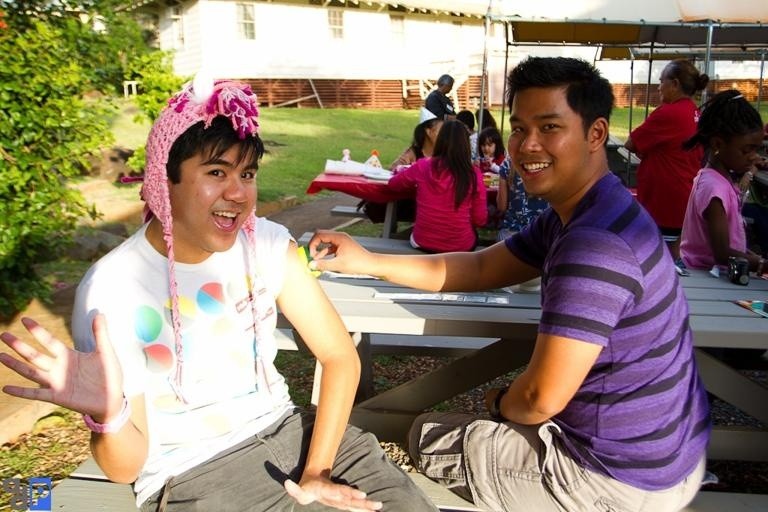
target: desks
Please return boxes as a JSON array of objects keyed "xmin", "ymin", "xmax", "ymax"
[
  {"xmin": 271, "ymin": 268, "xmax": 768, "ymax": 465},
  {"xmin": 309, "ymin": 169, "xmax": 499, "ymax": 241}
]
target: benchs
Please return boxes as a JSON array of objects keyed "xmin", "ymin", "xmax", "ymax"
[
  {"xmin": 23, "ymin": 455, "xmax": 767, "ymax": 510},
  {"xmin": 296, "ymin": 203, "xmax": 487, "ymax": 255}
]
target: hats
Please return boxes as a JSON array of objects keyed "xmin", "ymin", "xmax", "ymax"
[
  {"xmin": 342, "ymin": 148, "xmax": 351, "ymax": 163},
  {"xmin": 140, "ymin": 80, "xmax": 260, "ymax": 236},
  {"xmin": 419, "ymin": 106, "xmax": 438, "ymax": 125},
  {"xmin": 362, "ymin": 149, "xmax": 383, "ymax": 175}
]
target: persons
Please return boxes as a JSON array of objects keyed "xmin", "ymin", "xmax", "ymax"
[
  {"xmin": 0, "ymin": 87, "xmax": 440, "ymax": 512},
  {"xmin": 309, "ymin": 58, "xmax": 720, "ymax": 512},
  {"xmin": 731, "ymin": 150, "xmax": 768, "ymax": 253},
  {"xmin": 388, "ymin": 73, "xmax": 552, "ymax": 255},
  {"xmin": 625, "ymin": 60, "xmax": 710, "ymax": 263},
  {"xmin": 674, "ymin": 89, "xmax": 765, "ymax": 271}
]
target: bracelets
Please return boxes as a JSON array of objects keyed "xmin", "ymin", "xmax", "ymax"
[
  {"xmin": 745, "ymin": 172, "xmax": 753, "ymax": 181},
  {"xmin": 493, "ymin": 387, "xmax": 509, "ymax": 420},
  {"xmin": 81, "ymin": 391, "xmax": 131, "ymax": 433}
]
